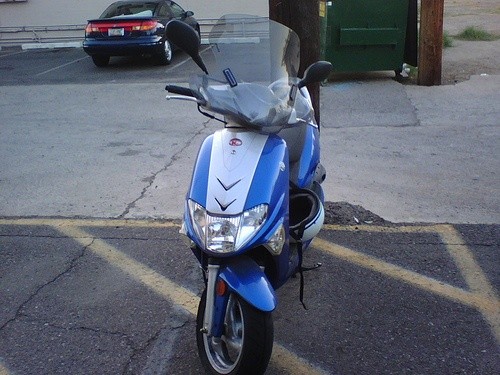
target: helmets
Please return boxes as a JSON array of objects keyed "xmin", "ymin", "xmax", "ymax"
[{"xmin": 288, "ymin": 179, "xmax": 325, "ymax": 310}]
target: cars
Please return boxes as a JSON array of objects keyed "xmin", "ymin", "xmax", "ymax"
[{"xmin": 81, "ymin": 0, "xmax": 202, "ymax": 69}]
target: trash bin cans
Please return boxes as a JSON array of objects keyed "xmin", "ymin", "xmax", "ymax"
[{"xmin": 320, "ymin": 1, "xmax": 417, "ymax": 88}]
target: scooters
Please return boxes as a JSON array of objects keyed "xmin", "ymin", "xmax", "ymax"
[{"xmin": 164, "ymin": 13, "xmax": 333, "ymax": 374}]
